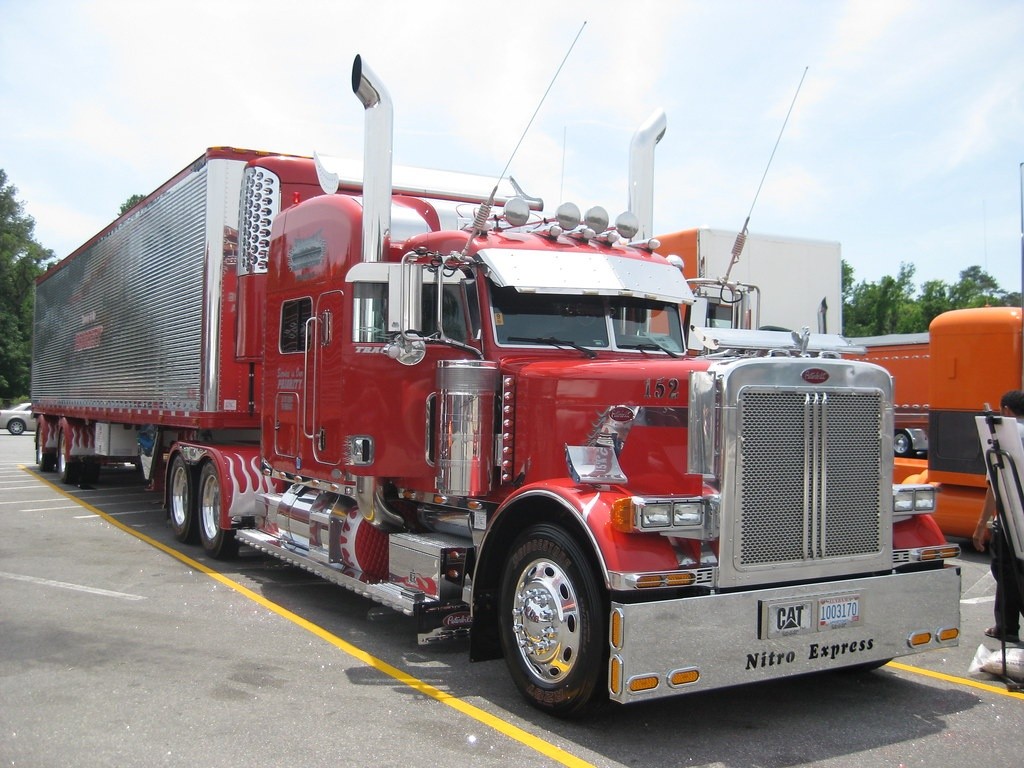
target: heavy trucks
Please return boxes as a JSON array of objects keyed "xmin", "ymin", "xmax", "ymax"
[{"xmin": 30, "ymin": 21, "xmax": 963, "ymax": 720}]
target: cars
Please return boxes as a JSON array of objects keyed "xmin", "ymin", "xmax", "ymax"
[{"xmin": 0, "ymin": 403, "xmax": 38, "ymax": 435}]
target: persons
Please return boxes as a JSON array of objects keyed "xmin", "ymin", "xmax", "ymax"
[{"xmin": 973, "ymin": 390, "xmax": 1024, "ymax": 642}]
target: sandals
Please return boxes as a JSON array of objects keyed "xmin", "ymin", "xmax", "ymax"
[{"xmin": 985, "ymin": 627, "xmax": 1020, "ymax": 643}]
